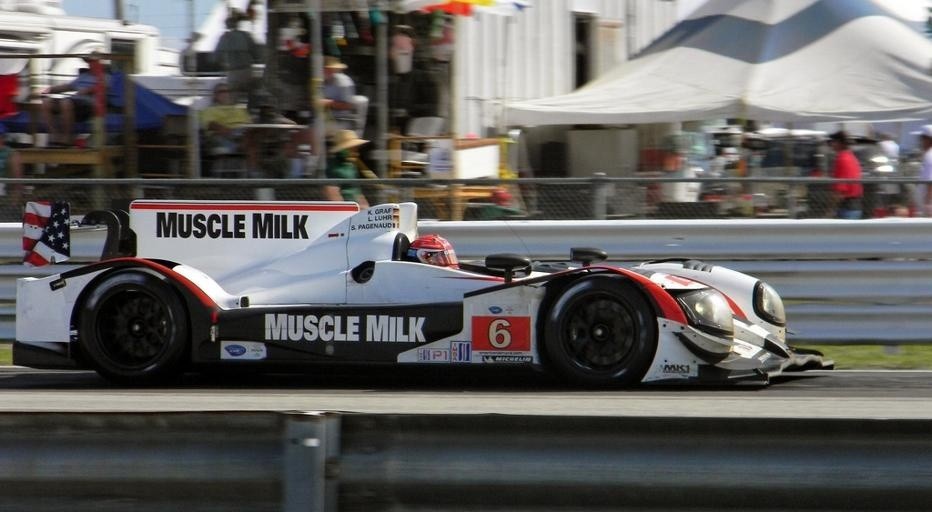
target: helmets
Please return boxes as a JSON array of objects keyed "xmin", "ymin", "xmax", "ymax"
[{"xmin": 405, "ymin": 233, "xmax": 461, "ymax": 271}]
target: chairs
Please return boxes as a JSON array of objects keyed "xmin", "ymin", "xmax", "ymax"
[{"xmin": 9, "ymin": 56, "xmax": 129, "ymax": 215}]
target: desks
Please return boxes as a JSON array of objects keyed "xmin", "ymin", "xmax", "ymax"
[{"xmin": 225, "ymin": 124, "xmax": 306, "ymax": 177}]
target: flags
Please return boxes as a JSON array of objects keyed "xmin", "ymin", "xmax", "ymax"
[{"xmin": 21, "ymin": 201, "xmax": 72, "ymax": 271}]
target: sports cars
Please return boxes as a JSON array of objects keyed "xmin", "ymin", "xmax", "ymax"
[{"xmin": 8, "ymin": 195, "xmax": 832, "ymax": 393}]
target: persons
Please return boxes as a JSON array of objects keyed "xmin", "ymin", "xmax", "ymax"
[
  {"xmin": 179, "ymin": 9, "xmax": 441, "ymax": 208},
  {"xmin": 823, "ymin": 129, "xmax": 864, "ymax": 220},
  {"xmin": 909, "ymin": 125, "xmax": 931, "ymax": 217},
  {"xmin": 39, "ymin": 49, "xmax": 115, "ymax": 149},
  {"xmin": 878, "ymin": 131, "xmax": 899, "ymax": 162}
]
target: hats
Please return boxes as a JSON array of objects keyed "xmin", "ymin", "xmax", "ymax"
[
  {"xmin": 81, "ymin": 51, "xmax": 100, "ymax": 61},
  {"xmin": 829, "ymin": 131, "xmax": 848, "ymax": 140},
  {"xmin": 318, "ymin": 56, "xmax": 348, "ymax": 69},
  {"xmin": 328, "ymin": 128, "xmax": 372, "ymax": 155},
  {"xmin": 909, "ymin": 124, "xmax": 932, "ymax": 138}
]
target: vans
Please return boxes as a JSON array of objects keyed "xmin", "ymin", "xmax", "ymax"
[{"xmin": 713, "ymin": 127, "xmax": 899, "ymax": 210}]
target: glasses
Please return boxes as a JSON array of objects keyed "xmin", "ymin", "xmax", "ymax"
[{"xmin": 216, "ymin": 89, "xmax": 231, "ymax": 94}]
